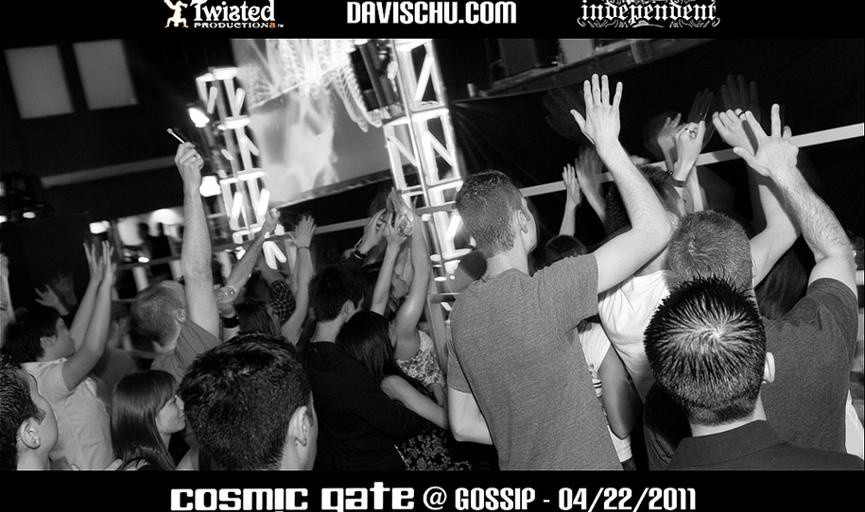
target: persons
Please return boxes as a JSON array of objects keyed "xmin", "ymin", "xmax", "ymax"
[{"xmin": 1, "ymin": 71, "xmax": 864, "ymax": 474}]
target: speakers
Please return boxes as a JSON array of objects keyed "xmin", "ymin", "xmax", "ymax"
[
  {"xmin": 348, "ymin": 40, "xmax": 397, "ymax": 112},
  {"xmin": 161, "ymin": 115, "xmax": 212, "ymax": 176}
]
